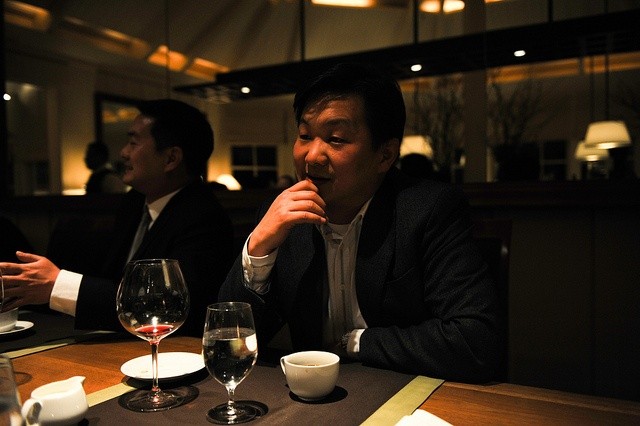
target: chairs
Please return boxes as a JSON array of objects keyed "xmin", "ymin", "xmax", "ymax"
[{"xmin": 476, "ymin": 219, "xmax": 513, "ymax": 376}]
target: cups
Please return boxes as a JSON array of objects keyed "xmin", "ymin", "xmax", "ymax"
[
  {"xmin": 0, "ymin": 307, "xmax": 19, "ymax": 332},
  {"xmin": 279, "ymin": 351, "xmax": 340, "ymax": 402},
  {"xmin": 0, "ymin": 354, "xmax": 28, "ymax": 426},
  {"xmin": 20, "ymin": 375, "xmax": 90, "ymax": 426}
]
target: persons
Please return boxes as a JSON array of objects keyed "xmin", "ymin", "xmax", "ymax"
[
  {"xmin": 82, "ymin": 140, "xmax": 128, "ymax": 194},
  {"xmin": 218, "ymin": 63, "xmax": 501, "ymax": 386},
  {"xmin": 1, "ymin": 98, "xmax": 243, "ymax": 333}
]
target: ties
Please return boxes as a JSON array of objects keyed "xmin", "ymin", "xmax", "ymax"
[{"xmin": 126, "ymin": 205, "xmax": 153, "ymax": 265}]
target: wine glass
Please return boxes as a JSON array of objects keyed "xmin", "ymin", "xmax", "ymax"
[
  {"xmin": 116, "ymin": 258, "xmax": 189, "ymax": 413},
  {"xmin": 201, "ymin": 302, "xmax": 258, "ymax": 425}
]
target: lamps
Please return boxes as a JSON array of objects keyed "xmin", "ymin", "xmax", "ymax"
[
  {"xmin": 574, "ymin": 57, "xmax": 609, "ymax": 162},
  {"xmin": 584, "ymin": 45, "xmax": 632, "ymax": 150}
]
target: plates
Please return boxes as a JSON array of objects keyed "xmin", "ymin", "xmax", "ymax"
[
  {"xmin": 120, "ymin": 351, "xmax": 205, "ymax": 381},
  {"xmin": 0, "ymin": 320, "xmax": 35, "ymax": 335}
]
